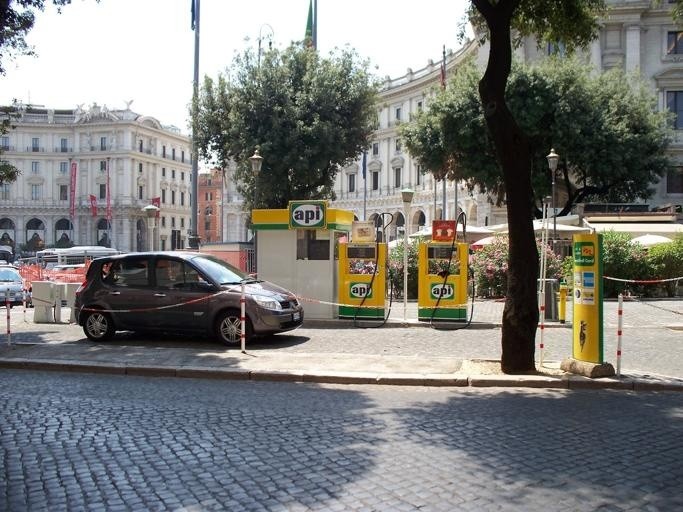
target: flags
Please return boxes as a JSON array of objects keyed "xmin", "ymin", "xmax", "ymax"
[
  {"xmin": 191, "ymin": 1, "xmax": 195, "ymax": 34},
  {"xmin": 547, "ymin": 41, "xmax": 552, "ymax": 57},
  {"xmin": 303, "ymin": 1, "xmax": 312, "ymax": 44},
  {"xmin": 361, "ymin": 151, "xmax": 365, "ymax": 179},
  {"xmin": 438, "ymin": 65, "xmax": 445, "ymax": 85}
]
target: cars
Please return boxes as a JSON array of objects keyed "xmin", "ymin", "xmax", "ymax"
[
  {"xmin": 0, "ymin": 268, "xmax": 27, "ymax": 305},
  {"xmin": 163, "ymin": 277, "xmax": 210, "ymax": 304},
  {"xmin": 73, "ymin": 250, "xmax": 303, "ymax": 345},
  {"xmin": 52, "ymin": 265, "xmax": 77, "ymax": 272}
]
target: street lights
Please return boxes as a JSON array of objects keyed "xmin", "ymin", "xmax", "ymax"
[
  {"xmin": 398, "ymin": 189, "xmax": 413, "ymax": 317},
  {"xmin": 249, "ymin": 146, "xmax": 263, "ymax": 276},
  {"xmin": 545, "ymin": 147, "xmax": 562, "ymax": 256}
]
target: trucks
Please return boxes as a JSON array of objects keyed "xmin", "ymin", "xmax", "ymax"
[
  {"xmin": 14, "ymin": 257, "xmax": 35, "ymax": 266},
  {"xmin": 0, "ymin": 246, "xmax": 12, "ymax": 267}
]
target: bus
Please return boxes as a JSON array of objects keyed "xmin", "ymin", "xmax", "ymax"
[{"xmin": 34, "ymin": 246, "xmax": 117, "ymax": 270}]
